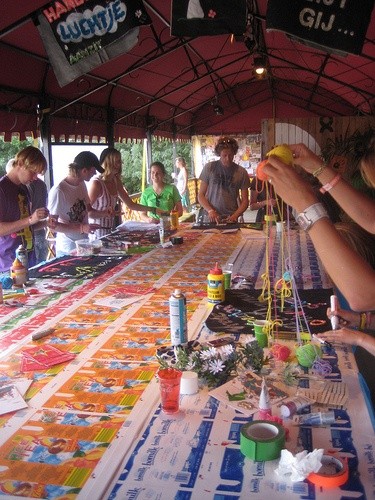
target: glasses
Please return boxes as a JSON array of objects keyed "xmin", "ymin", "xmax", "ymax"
[
  {"xmin": 219, "ymin": 139, "xmax": 232, "ymax": 144},
  {"xmin": 156, "ymin": 195, "xmax": 162, "ymax": 208}
]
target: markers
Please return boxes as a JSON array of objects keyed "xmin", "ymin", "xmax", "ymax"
[
  {"xmin": 312, "ymin": 334, "xmax": 332, "ymax": 348},
  {"xmin": 32, "ymin": 328, "xmax": 56, "ymax": 341}
]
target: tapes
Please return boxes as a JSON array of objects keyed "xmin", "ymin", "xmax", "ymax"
[
  {"xmin": 180, "ymin": 370, "xmax": 198, "ymax": 396},
  {"xmin": 306, "ymin": 452, "xmax": 349, "ymax": 488},
  {"xmin": 239, "ymin": 419, "xmax": 286, "ymax": 464}
]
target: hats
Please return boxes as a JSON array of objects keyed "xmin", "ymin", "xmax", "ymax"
[
  {"xmin": 100, "ymin": 148, "xmax": 119, "ymax": 163},
  {"xmin": 73, "ymin": 151, "xmax": 106, "ymax": 173}
]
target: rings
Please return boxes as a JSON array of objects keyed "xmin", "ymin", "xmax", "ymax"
[{"xmin": 269, "ymin": 178, "xmax": 272, "ymax": 183}]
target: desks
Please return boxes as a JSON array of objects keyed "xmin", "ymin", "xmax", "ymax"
[{"xmin": 0, "ymin": 222, "xmax": 375, "ymax": 500}]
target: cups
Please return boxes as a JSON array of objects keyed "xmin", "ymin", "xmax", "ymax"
[
  {"xmin": 158, "ymin": 369, "xmax": 182, "ymax": 414},
  {"xmin": 253, "ymin": 320, "xmax": 272, "ymax": 347},
  {"xmin": 223, "ymin": 273, "xmax": 231, "ymax": 289},
  {"xmin": 276, "ymin": 222, "xmax": 286, "ymax": 232}
]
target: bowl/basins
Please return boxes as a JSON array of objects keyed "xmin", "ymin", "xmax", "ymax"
[{"xmin": 74, "ymin": 240, "xmax": 101, "ymax": 254}]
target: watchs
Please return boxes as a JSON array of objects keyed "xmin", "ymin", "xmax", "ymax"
[{"xmin": 295, "ymin": 202, "xmax": 330, "ymax": 231}]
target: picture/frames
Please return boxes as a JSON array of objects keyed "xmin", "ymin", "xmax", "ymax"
[{"xmin": 75, "ymin": 239, "xmax": 103, "ymax": 256}]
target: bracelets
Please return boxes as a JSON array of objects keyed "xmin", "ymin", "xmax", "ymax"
[
  {"xmin": 313, "ymin": 162, "xmax": 327, "ymax": 176},
  {"xmin": 360, "ymin": 312, "xmax": 371, "ymax": 330},
  {"xmin": 319, "ymin": 174, "xmax": 341, "ymax": 194}
]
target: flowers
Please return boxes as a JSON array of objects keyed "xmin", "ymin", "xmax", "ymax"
[{"xmin": 178, "ymin": 342, "xmax": 263, "ymax": 388}]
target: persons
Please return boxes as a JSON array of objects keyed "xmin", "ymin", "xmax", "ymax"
[
  {"xmin": 360, "ymin": 149, "xmax": 375, "ymax": 204},
  {"xmin": 138, "ymin": 162, "xmax": 183, "ymax": 224},
  {"xmin": 250, "ymin": 175, "xmax": 293, "ymax": 223},
  {"xmin": 262, "ymin": 143, "xmax": 375, "ymax": 312},
  {"xmin": 6, "ymin": 159, "xmax": 48, "ymax": 266},
  {"xmin": 88, "ymin": 148, "xmax": 168, "ymax": 241},
  {"xmin": 197, "ymin": 136, "xmax": 251, "ymax": 224},
  {"xmin": 48, "ymin": 151, "xmax": 105, "ymax": 258},
  {"xmin": 175, "ymin": 157, "xmax": 190, "ymax": 208},
  {"xmin": 314, "ymin": 223, "xmax": 375, "ymax": 392},
  {"xmin": 0, "ymin": 146, "xmax": 49, "ymax": 275}
]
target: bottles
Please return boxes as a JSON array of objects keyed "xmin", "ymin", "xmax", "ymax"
[
  {"xmin": 16, "ymin": 244, "xmax": 30, "ymax": 280},
  {"xmin": 169, "ymin": 289, "xmax": 188, "ymax": 345},
  {"xmin": 10, "ymin": 258, "xmax": 26, "ymax": 286},
  {"xmin": 170, "ymin": 210, "xmax": 178, "ymax": 227},
  {"xmin": 206, "ymin": 261, "xmax": 225, "ymax": 303}
]
